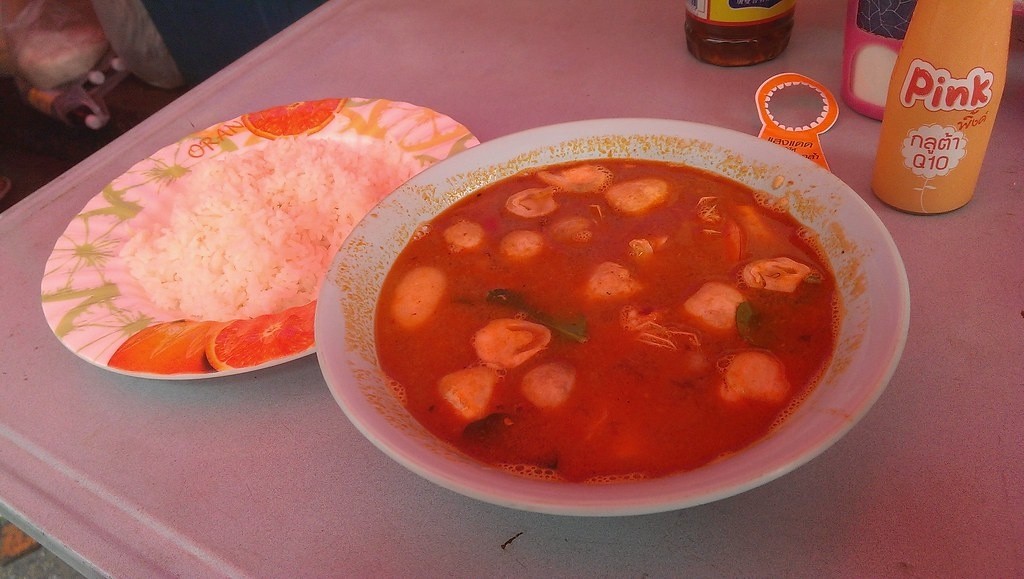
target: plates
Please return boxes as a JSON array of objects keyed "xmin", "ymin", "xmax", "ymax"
[
  {"xmin": 312, "ymin": 119, "xmax": 914, "ymax": 518},
  {"xmin": 38, "ymin": 93, "xmax": 482, "ymax": 380}
]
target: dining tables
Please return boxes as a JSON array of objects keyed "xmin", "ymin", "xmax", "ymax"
[{"xmin": 0, "ymin": 0, "xmax": 1024, "ymax": 578}]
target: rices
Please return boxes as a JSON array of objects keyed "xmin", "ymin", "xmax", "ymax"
[{"xmin": 120, "ymin": 136, "xmax": 414, "ymax": 323}]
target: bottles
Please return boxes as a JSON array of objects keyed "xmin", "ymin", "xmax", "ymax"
[
  {"xmin": 870, "ymin": 2, "xmax": 1014, "ymax": 216},
  {"xmin": 683, "ymin": 0, "xmax": 796, "ymax": 68}
]
